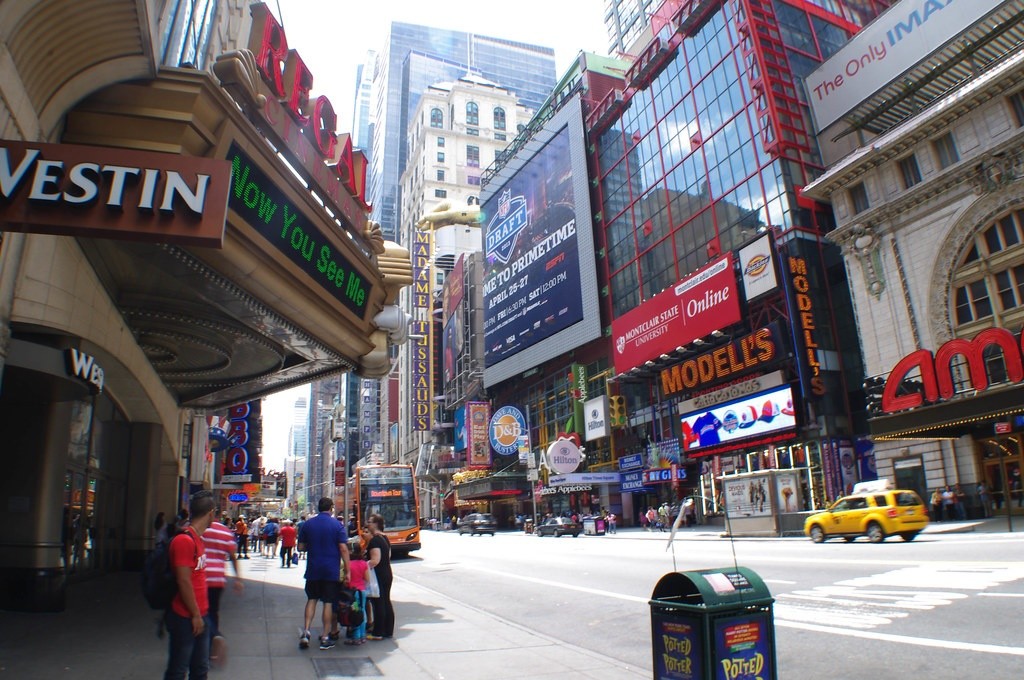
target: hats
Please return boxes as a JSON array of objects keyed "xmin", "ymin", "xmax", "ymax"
[{"xmin": 239, "ymin": 515, "xmax": 246, "ymax": 518}]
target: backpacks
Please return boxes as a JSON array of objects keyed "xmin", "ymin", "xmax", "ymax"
[{"xmin": 134, "ymin": 531, "xmax": 198, "ymax": 610}]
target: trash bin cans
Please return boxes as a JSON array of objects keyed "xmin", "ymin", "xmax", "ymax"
[
  {"xmin": 525, "ymin": 518, "xmax": 533, "ymax": 534},
  {"xmin": 583, "ymin": 516, "xmax": 605, "ymax": 536},
  {"xmin": 648, "ymin": 567, "xmax": 777, "ymax": 680}
]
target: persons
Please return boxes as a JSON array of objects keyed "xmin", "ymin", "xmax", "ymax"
[
  {"xmin": 508, "ymin": 507, "xmax": 616, "ymax": 534},
  {"xmin": 929, "ymin": 480, "xmax": 992, "ymax": 522},
  {"xmin": 639, "ymin": 502, "xmax": 690, "ymax": 532},
  {"xmin": 220, "ymin": 498, "xmax": 407, "ymax": 569},
  {"xmin": 153, "ymin": 490, "xmax": 239, "ymax": 680},
  {"xmin": 296, "ymin": 497, "xmax": 395, "ymax": 650},
  {"xmin": 426, "ymin": 513, "xmax": 463, "ymax": 530},
  {"xmin": 802, "ymin": 490, "xmax": 843, "ymax": 510}
]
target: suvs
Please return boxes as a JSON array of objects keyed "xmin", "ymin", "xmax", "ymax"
[{"xmin": 458, "ymin": 513, "xmax": 497, "ymax": 536}]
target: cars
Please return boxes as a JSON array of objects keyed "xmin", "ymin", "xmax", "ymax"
[
  {"xmin": 534, "ymin": 515, "xmax": 585, "ymax": 538},
  {"xmin": 804, "ymin": 479, "xmax": 931, "ymax": 544}
]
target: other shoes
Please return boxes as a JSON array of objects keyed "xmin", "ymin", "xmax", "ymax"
[
  {"xmin": 299, "ymin": 632, "xmax": 310, "ymax": 648},
  {"xmin": 343, "ymin": 638, "xmax": 365, "ymax": 645},
  {"xmin": 367, "ymin": 634, "xmax": 382, "ymax": 641},
  {"xmin": 244, "ymin": 555, "xmax": 249, "ymax": 559},
  {"xmin": 319, "ymin": 631, "xmax": 339, "ymax": 640},
  {"xmin": 238, "ymin": 555, "xmax": 242, "ymax": 558},
  {"xmin": 366, "ymin": 622, "xmax": 373, "ymax": 633},
  {"xmin": 209, "ymin": 636, "xmax": 224, "ymax": 660},
  {"xmin": 320, "ymin": 637, "xmax": 335, "ymax": 650}
]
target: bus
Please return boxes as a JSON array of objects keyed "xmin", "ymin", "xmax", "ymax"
[{"xmin": 333, "ymin": 463, "xmax": 422, "ymax": 557}]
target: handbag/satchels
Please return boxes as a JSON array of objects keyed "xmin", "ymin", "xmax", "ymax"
[
  {"xmin": 262, "ymin": 534, "xmax": 268, "ymax": 539},
  {"xmin": 338, "ymin": 589, "xmax": 364, "ymax": 627},
  {"xmin": 292, "ymin": 553, "xmax": 298, "ymax": 564},
  {"xmin": 365, "ymin": 561, "xmax": 379, "ymax": 597}
]
